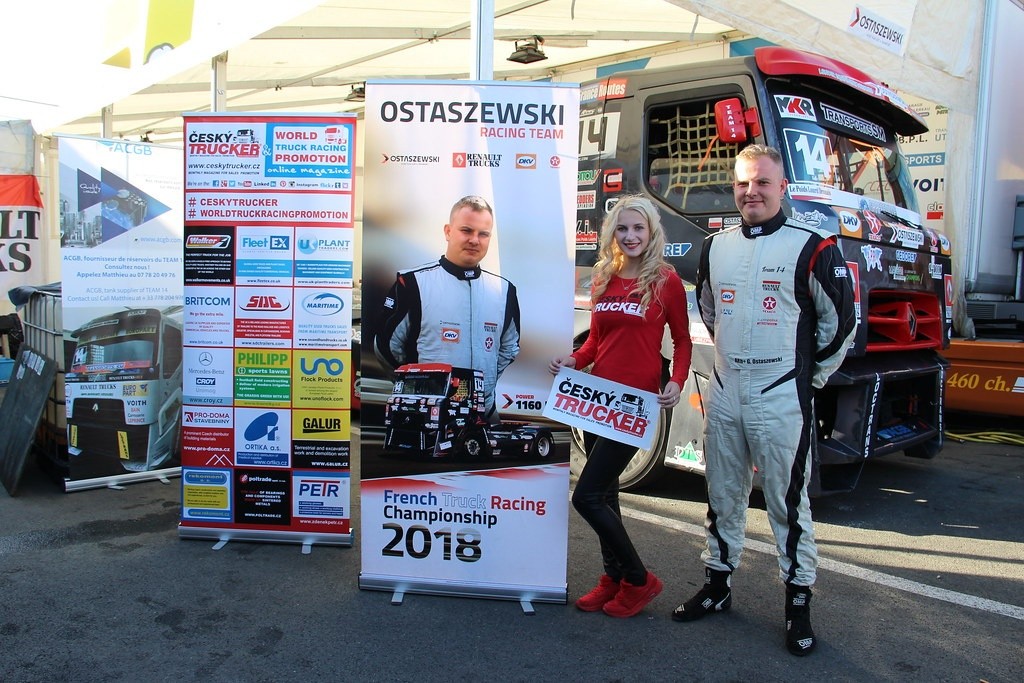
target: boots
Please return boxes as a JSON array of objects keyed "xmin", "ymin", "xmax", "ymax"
[
  {"xmin": 670, "ymin": 568, "xmax": 732, "ymax": 623},
  {"xmin": 781, "ymin": 583, "xmax": 816, "ymax": 657}
]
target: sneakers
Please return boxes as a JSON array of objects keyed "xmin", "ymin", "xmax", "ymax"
[
  {"xmin": 575, "ymin": 574, "xmax": 621, "ymax": 613},
  {"xmin": 602, "ymin": 569, "xmax": 664, "ymax": 618}
]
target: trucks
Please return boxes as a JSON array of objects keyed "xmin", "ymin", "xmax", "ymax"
[
  {"xmin": 576, "ymin": 43, "xmax": 955, "ymax": 507},
  {"xmin": 384, "ymin": 361, "xmax": 555, "ymax": 466},
  {"xmin": 63, "ymin": 308, "xmax": 182, "ymax": 472}
]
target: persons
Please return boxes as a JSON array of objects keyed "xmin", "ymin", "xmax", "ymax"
[
  {"xmin": 671, "ymin": 144, "xmax": 856, "ymax": 657},
  {"xmin": 549, "ymin": 195, "xmax": 693, "ymax": 619},
  {"xmin": 373, "ymin": 197, "xmax": 521, "ymax": 430}
]
